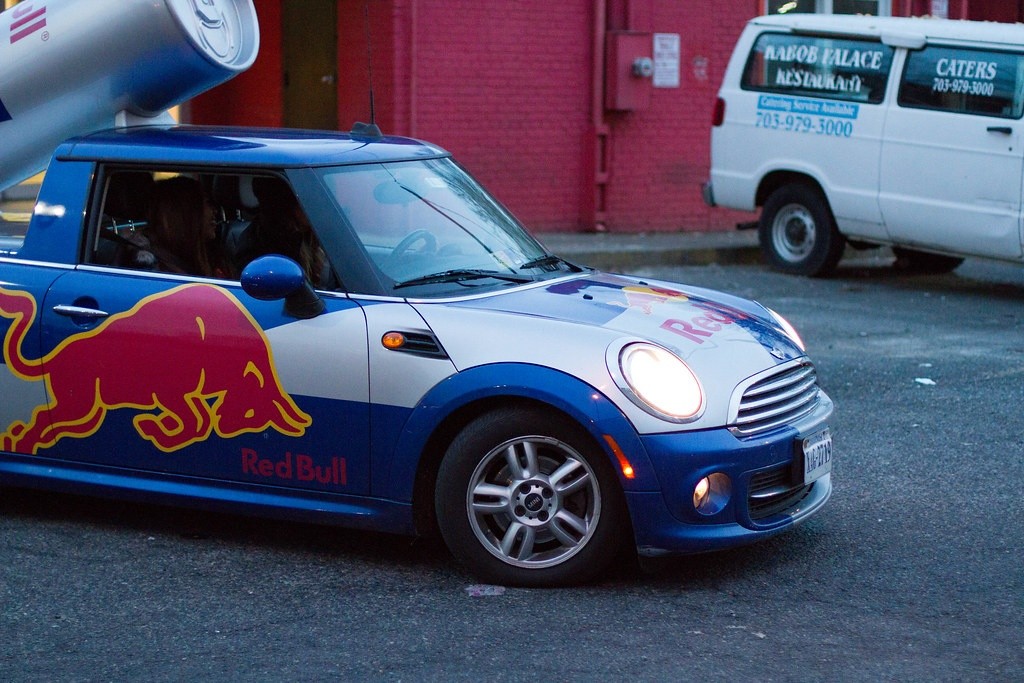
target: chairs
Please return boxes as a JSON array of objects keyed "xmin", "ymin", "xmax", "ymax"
[
  {"xmin": 221, "ymin": 173, "xmax": 302, "ymax": 277},
  {"xmin": 94, "ymin": 171, "xmax": 156, "ymax": 264}
]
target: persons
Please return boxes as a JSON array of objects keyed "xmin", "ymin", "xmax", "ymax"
[{"xmin": 101, "ymin": 172, "xmax": 340, "ymax": 292}]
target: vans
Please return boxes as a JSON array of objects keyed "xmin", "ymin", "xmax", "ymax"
[{"xmin": 702, "ymin": 15, "xmax": 1022, "ymax": 278}]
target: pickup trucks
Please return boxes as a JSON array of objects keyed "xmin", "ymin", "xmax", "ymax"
[{"xmin": 1, "ymin": 0, "xmax": 832, "ymax": 587}]
